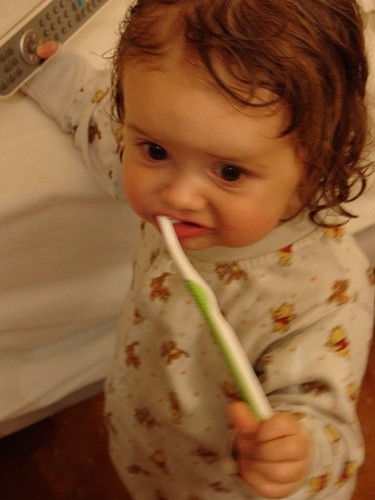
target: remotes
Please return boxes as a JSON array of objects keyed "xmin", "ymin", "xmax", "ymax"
[{"xmin": 0, "ymin": 0, "xmax": 123, "ymax": 100}]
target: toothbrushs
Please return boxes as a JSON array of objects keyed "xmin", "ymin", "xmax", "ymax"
[{"xmin": 157, "ymin": 214, "xmax": 276, "ymax": 424}]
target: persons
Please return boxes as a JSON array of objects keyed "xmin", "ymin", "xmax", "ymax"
[{"xmin": 19, "ymin": 0, "xmax": 374, "ymax": 499}]
